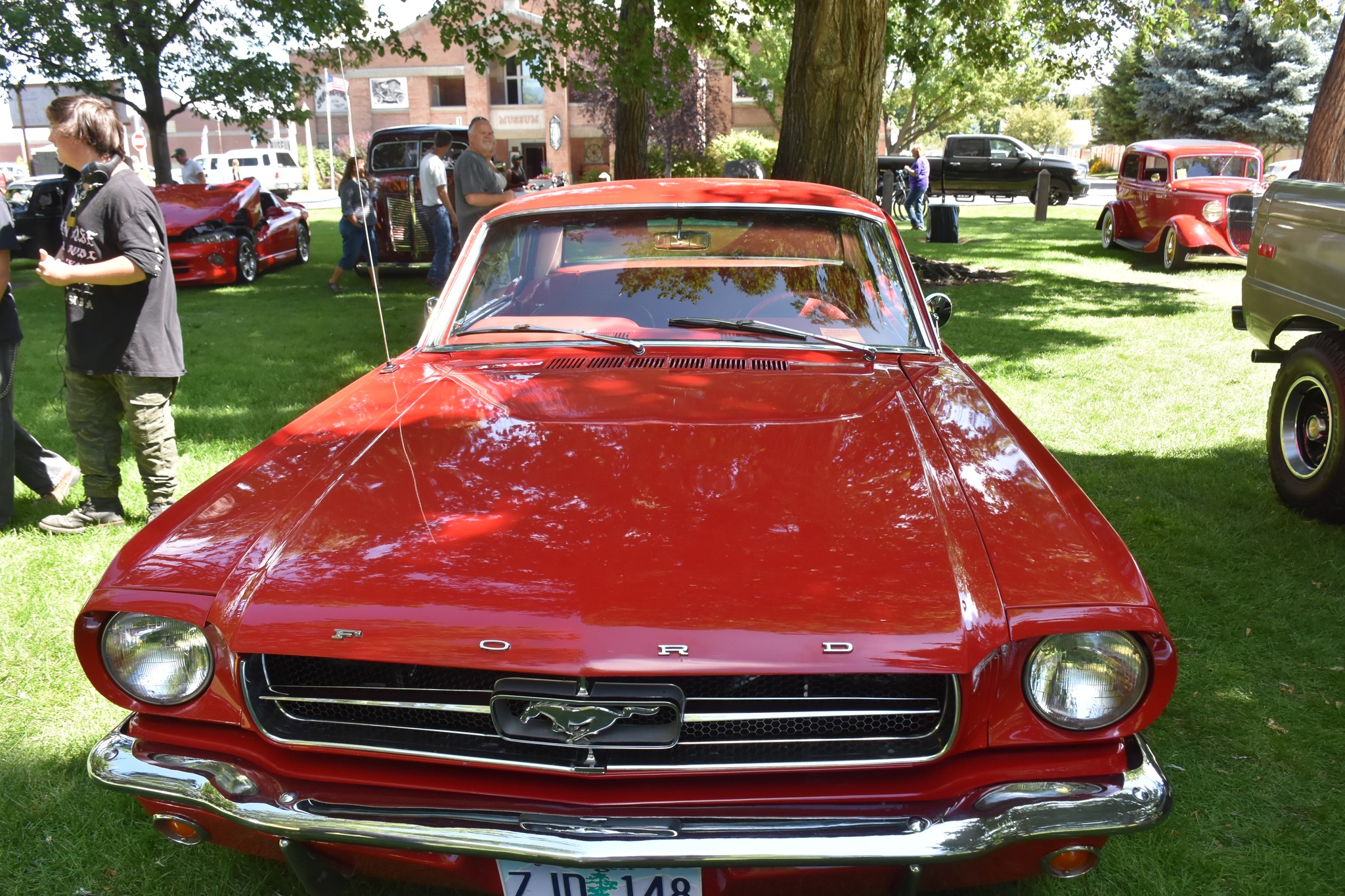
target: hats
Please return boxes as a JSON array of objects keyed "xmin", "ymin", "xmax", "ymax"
[
  {"xmin": 171, "ymin": 149, "xmax": 186, "ymax": 157},
  {"xmin": 512, "ymin": 154, "xmax": 524, "ymax": 162}
]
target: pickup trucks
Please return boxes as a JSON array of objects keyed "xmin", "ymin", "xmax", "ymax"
[
  {"xmin": 1231, "ymin": 176, "xmax": 1345, "ymax": 512},
  {"xmin": 878, "ymin": 134, "xmax": 1091, "ymax": 206}
]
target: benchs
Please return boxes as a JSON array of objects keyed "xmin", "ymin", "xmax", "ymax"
[{"xmin": 517, "ymin": 256, "xmax": 880, "ymax": 329}]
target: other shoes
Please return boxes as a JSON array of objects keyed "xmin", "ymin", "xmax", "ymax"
[
  {"xmin": 371, "ymin": 284, "xmax": 387, "ymax": 291},
  {"xmin": 910, "ymin": 223, "xmax": 921, "ymax": 230},
  {"xmin": 31, "ymin": 464, "xmax": 81, "ymax": 508},
  {"xmin": 425, "ymin": 277, "xmax": 445, "ymax": 285},
  {"xmin": 327, "ymin": 280, "xmax": 343, "ymax": 294}
]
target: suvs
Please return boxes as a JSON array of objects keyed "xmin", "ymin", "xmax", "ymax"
[
  {"xmin": 355, "ymin": 125, "xmax": 508, "ymax": 263},
  {"xmin": 180, "ymin": 148, "xmax": 304, "ymax": 200}
]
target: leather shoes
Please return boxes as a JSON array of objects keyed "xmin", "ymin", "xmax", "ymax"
[
  {"xmin": 147, "ymin": 496, "xmax": 177, "ymax": 523},
  {"xmin": 39, "ymin": 495, "xmax": 126, "ymax": 535}
]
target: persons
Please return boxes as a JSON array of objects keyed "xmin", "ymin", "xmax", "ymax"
[
  {"xmin": 231, "ymin": 158, "xmax": 241, "ymax": 182},
  {"xmin": 0, "ymin": 193, "xmax": 81, "ymax": 525},
  {"xmin": 327, "ymin": 158, "xmax": 382, "ymax": 293},
  {"xmin": 506, "ymin": 154, "xmax": 529, "ymax": 192},
  {"xmin": 453, "ymin": 116, "xmax": 516, "ymax": 311},
  {"xmin": 904, "ymin": 144, "xmax": 930, "ymax": 230},
  {"xmin": 38, "ymin": 95, "xmax": 191, "ymax": 535},
  {"xmin": 418, "ymin": 130, "xmax": 458, "ymax": 287},
  {"xmin": 173, "ymin": 147, "xmax": 205, "ymax": 185}
]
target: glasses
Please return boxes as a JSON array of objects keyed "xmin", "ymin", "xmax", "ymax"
[{"xmin": 471, "ymin": 117, "xmax": 489, "ymax": 124}]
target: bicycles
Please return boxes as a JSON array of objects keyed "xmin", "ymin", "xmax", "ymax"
[{"xmin": 876, "ymin": 169, "xmax": 931, "ymax": 220}]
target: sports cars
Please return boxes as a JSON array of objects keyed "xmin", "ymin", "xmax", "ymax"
[{"xmin": 149, "ymin": 178, "xmax": 311, "ymax": 290}]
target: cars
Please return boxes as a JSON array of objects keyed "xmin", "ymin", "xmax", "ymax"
[
  {"xmin": 5, "ymin": 174, "xmax": 74, "ymax": 245},
  {"xmin": 74, "ymin": 174, "xmax": 1179, "ymax": 894},
  {"xmin": 1263, "ymin": 158, "xmax": 1303, "ymax": 181},
  {"xmin": 1094, "ymin": 139, "xmax": 1278, "ymax": 274}
]
